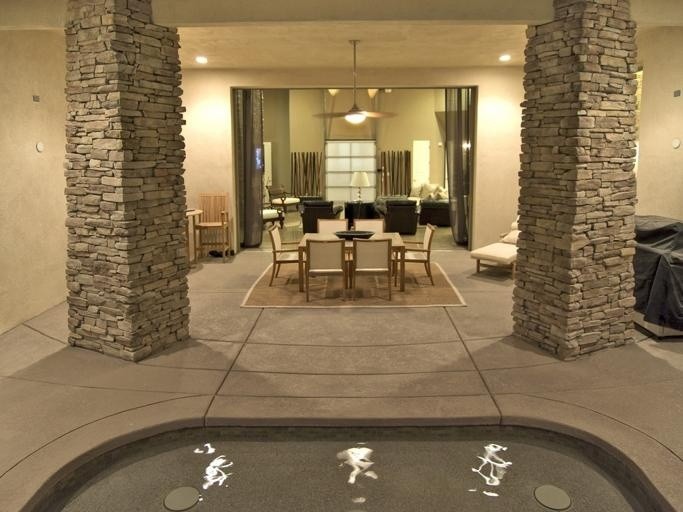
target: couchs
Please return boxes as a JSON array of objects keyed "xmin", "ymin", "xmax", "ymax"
[{"xmin": 420, "ymin": 199, "xmax": 450, "ymax": 227}]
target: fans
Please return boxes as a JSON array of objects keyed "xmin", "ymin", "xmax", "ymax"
[{"xmin": 313, "ymin": 40, "xmax": 398, "ymax": 120}]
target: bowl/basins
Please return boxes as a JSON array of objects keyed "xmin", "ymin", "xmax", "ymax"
[{"xmin": 335, "ymin": 231, "xmax": 375, "ymax": 241}]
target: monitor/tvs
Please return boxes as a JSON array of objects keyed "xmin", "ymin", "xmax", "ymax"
[{"xmin": 252, "ymin": 144, "xmax": 264, "ymax": 175}]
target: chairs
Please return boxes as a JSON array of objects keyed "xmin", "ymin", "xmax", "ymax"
[
  {"xmin": 266, "ymin": 185, "xmax": 301, "ymax": 212},
  {"xmin": 376, "ymin": 197, "xmax": 416, "ymax": 234},
  {"xmin": 263, "ymin": 208, "xmax": 284, "ymax": 229},
  {"xmin": 301, "ymin": 202, "xmax": 343, "ymax": 233},
  {"xmin": 195, "ymin": 193, "xmax": 231, "ymax": 263},
  {"xmin": 470, "ymin": 216, "xmax": 521, "ymax": 280},
  {"xmin": 345, "ymin": 204, "xmax": 380, "ymax": 229},
  {"xmin": 268, "ymin": 219, "xmax": 435, "ymax": 302}
]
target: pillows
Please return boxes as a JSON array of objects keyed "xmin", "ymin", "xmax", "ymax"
[{"xmin": 409, "ymin": 181, "xmax": 448, "ymax": 200}]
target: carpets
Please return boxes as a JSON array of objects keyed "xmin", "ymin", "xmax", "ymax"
[{"xmin": 240, "ymin": 262, "xmax": 467, "ymax": 308}]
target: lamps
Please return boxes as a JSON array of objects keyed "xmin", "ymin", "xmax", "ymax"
[
  {"xmin": 343, "ymin": 113, "xmax": 365, "ymax": 124},
  {"xmin": 349, "ymin": 172, "xmax": 371, "ymax": 201}
]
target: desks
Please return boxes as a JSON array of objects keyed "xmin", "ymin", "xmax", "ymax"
[{"xmin": 186, "ymin": 209, "xmax": 203, "ymax": 267}]
